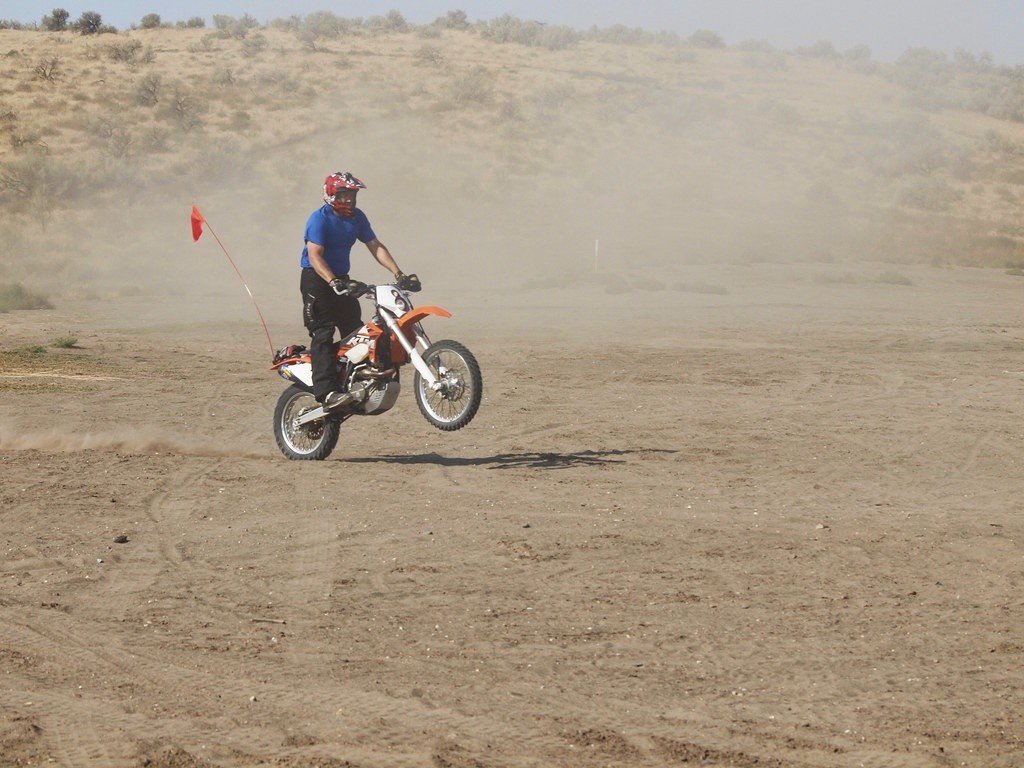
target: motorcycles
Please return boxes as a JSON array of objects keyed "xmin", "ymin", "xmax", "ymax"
[{"xmin": 270, "ymin": 277, "xmax": 481, "ymax": 462}]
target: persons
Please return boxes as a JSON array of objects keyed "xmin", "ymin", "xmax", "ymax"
[{"xmin": 300, "ymin": 171, "xmax": 420, "ymax": 411}]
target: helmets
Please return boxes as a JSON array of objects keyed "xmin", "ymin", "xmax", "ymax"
[{"xmin": 323, "ymin": 172, "xmax": 367, "ymax": 217}]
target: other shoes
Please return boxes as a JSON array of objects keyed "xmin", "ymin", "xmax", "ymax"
[{"xmin": 321, "ymin": 390, "xmax": 354, "ymax": 413}]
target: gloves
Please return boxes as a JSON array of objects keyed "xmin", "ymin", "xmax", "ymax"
[
  {"xmin": 395, "ymin": 274, "xmax": 422, "ymax": 292},
  {"xmin": 329, "ymin": 275, "xmax": 367, "ymax": 297}
]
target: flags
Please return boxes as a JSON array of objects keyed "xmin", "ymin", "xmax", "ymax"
[{"xmin": 191, "ymin": 206, "xmax": 203, "ymax": 242}]
